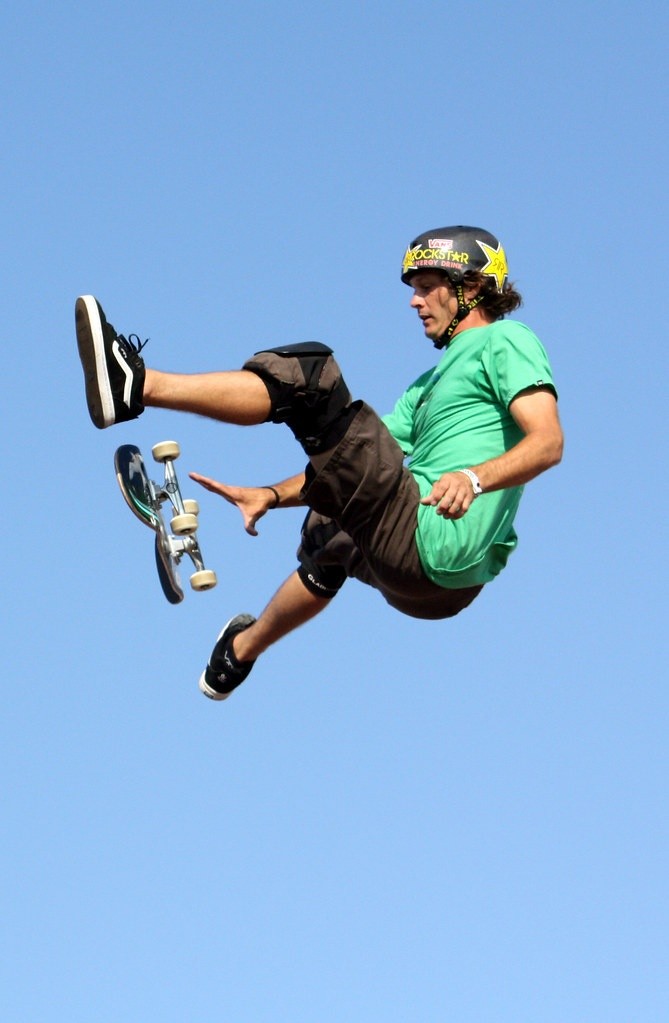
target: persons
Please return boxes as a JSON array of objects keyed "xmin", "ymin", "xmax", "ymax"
[{"xmin": 74, "ymin": 226, "xmax": 564, "ymax": 705}]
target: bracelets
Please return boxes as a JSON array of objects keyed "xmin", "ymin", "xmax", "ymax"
[
  {"xmin": 261, "ymin": 485, "xmax": 280, "ymax": 509},
  {"xmin": 458, "ymin": 469, "xmax": 483, "ymax": 499}
]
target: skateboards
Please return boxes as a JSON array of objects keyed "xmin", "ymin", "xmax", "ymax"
[{"xmin": 112, "ymin": 439, "xmax": 218, "ymax": 605}]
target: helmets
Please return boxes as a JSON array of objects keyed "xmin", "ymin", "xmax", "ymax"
[{"xmin": 400, "ymin": 225, "xmax": 509, "ymax": 296}]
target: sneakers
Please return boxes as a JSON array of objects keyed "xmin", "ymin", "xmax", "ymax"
[
  {"xmin": 199, "ymin": 613, "xmax": 257, "ymax": 701},
  {"xmin": 75, "ymin": 295, "xmax": 149, "ymax": 429}
]
